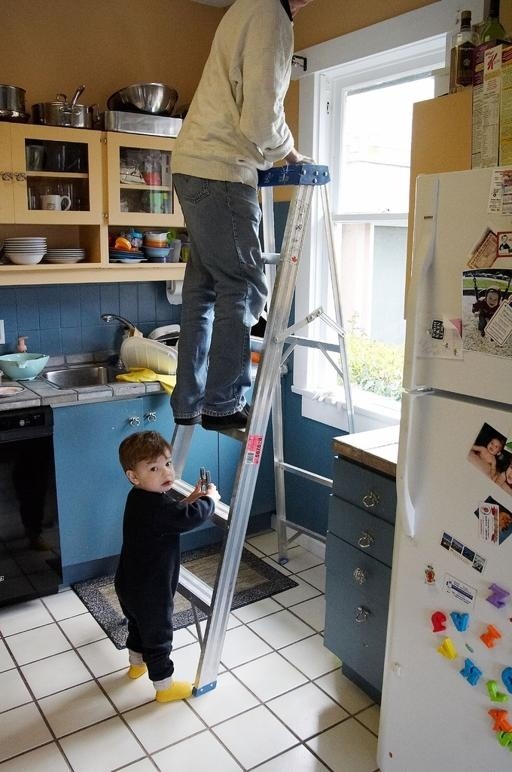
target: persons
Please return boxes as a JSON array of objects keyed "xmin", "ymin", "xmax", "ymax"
[
  {"xmin": 113, "ymin": 430, "xmax": 221, "ymax": 703},
  {"xmin": 170, "ymin": 0, "xmax": 314, "ymax": 430},
  {"xmin": 471, "ymin": 437, "xmax": 504, "ymax": 478},
  {"xmin": 496, "ymin": 459, "xmax": 512, "ymax": 487},
  {"xmin": 473, "ymin": 289, "xmax": 501, "ymax": 341}
]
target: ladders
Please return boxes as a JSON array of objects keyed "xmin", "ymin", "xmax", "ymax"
[{"xmin": 119, "ymin": 162, "xmax": 356, "ymax": 697}]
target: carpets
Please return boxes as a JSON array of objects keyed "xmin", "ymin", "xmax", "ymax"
[{"xmin": 70, "ymin": 541, "xmax": 299, "ymax": 650}]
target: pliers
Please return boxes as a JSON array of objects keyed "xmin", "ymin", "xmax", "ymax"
[{"xmin": 200, "ymin": 467, "xmax": 211, "ymax": 496}]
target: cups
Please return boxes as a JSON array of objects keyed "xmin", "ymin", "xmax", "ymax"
[
  {"xmin": 180, "ymin": 246, "xmax": 191, "ymax": 262},
  {"xmin": 28, "ymin": 182, "xmax": 82, "ymax": 211},
  {"xmin": 25, "ymin": 144, "xmax": 88, "ymax": 173}
]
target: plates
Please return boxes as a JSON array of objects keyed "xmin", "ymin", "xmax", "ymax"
[
  {"xmin": 3, "ymin": 236, "xmax": 85, "ymax": 265},
  {"xmin": 0, "ymin": 385, "xmax": 27, "ymax": 398},
  {"xmin": 109, "ymin": 249, "xmax": 148, "ymax": 264}
]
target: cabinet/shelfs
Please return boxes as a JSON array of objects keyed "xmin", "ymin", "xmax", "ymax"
[
  {"xmin": 219, "ymin": 380, "xmax": 276, "ymax": 535},
  {"xmin": 323, "ymin": 422, "xmax": 400, "ymax": 706},
  {"xmin": 52, "ymin": 390, "xmax": 219, "ymax": 589},
  {"xmin": 0, "ymin": 120, "xmax": 187, "ymax": 287}
]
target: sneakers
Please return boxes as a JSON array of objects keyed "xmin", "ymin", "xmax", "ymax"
[
  {"xmin": 173, "ymin": 413, "xmax": 202, "ymax": 426},
  {"xmin": 127, "ymin": 664, "xmax": 147, "ymax": 679},
  {"xmin": 201, "ymin": 403, "xmax": 251, "ymax": 430},
  {"xmin": 156, "ymin": 680, "xmax": 192, "ymax": 703}
]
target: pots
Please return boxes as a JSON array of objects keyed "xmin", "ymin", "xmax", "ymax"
[
  {"xmin": 0, "ymin": 84, "xmax": 31, "ymax": 122},
  {"xmin": 104, "ymin": 82, "xmax": 179, "ymax": 117},
  {"xmin": 99, "ymin": 110, "xmax": 183, "ymax": 138}
]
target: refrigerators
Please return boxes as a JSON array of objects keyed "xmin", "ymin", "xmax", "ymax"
[{"xmin": 376, "ymin": 165, "xmax": 512, "ymax": 772}]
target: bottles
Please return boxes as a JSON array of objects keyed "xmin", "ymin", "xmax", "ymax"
[{"xmin": 449, "ymin": 0, "xmax": 507, "ymax": 94}]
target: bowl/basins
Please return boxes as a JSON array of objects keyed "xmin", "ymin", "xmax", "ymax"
[
  {"xmin": 1, "ymin": 352, "xmax": 50, "ymax": 382},
  {"xmin": 31, "ymin": 84, "xmax": 99, "ymax": 130},
  {"xmin": 142, "ymin": 230, "xmax": 174, "ymax": 263}
]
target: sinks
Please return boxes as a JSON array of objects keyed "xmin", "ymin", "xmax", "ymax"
[{"xmin": 37, "ymin": 362, "xmax": 129, "ymax": 390}]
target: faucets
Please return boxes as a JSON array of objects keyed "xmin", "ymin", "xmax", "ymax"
[{"xmin": 101, "ymin": 314, "xmax": 135, "ymax": 329}]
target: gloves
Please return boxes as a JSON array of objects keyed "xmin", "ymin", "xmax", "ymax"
[{"xmin": 116, "ymin": 367, "xmax": 177, "ymax": 396}]
